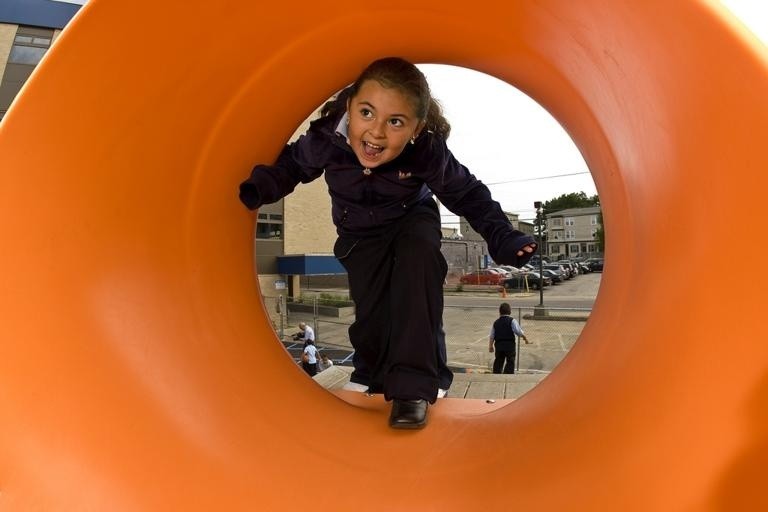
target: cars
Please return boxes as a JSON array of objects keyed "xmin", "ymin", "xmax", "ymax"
[{"xmin": 460, "ymin": 255, "xmax": 604, "ymax": 290}]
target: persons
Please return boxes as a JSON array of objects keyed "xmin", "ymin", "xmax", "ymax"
[
  {"xmin": 298, "ymin": 319, "xmax": 335, "ymax": 377},
  {"xmin": 488, "ymin": 303, "xmax": 532, "ymax": 374},
  {"xmin": 239, "ymin": 55, "xmax": 538, "ymax": 429}
]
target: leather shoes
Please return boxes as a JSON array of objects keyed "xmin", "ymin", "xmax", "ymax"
[{"xmin": 389, "ymin": 398, "xmax": 429, "ymax": 429}]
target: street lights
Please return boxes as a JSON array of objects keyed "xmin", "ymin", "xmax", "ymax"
[{"xmin": 533, "ymin": 201, "xmax": 543, "ymax": 304}]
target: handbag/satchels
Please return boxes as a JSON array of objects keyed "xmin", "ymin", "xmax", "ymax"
[{"xmin": 301, "ymin": 353, "xmax": 309, "ymax": 363}]
